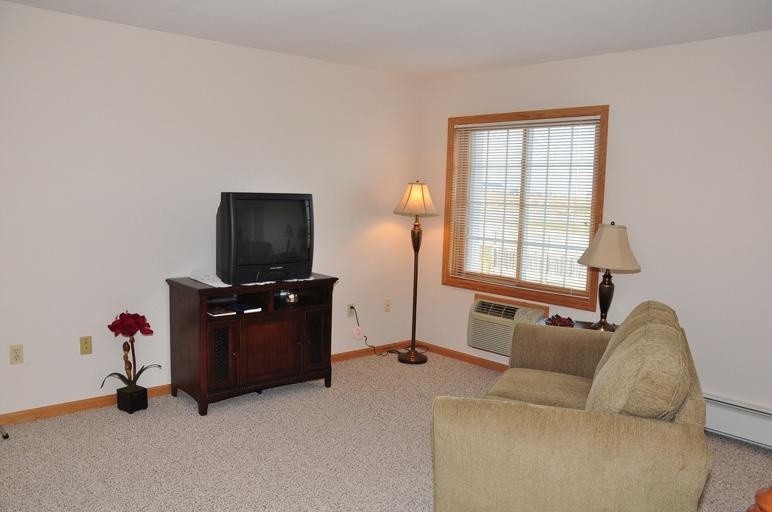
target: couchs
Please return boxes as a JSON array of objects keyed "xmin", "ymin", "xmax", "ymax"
[{"xmin": 430, "ymin": 301, "xmax": 711, "ymax": 512}]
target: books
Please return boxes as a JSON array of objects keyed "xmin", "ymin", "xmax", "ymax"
[
  {"xmin": 227, "ymin": 305, "xmax": 262, "ymax": 314},
  {"xmin": 207, "ymin": 308, "xmax": 237, "ymax": 317}
]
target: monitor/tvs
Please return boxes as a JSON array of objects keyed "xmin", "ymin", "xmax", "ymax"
[{"xmin": 216, "ymin": 192, "xmax": 313, "ymax": 287}]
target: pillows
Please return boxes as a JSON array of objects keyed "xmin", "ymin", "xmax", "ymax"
[{"xmin": 584, "ymin": 301, "xmax": 692, "ymax": 421}]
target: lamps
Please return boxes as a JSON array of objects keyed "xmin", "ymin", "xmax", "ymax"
[
  {"xmin": 394, "ymin": 180, "xmax": 440, "ymax": 365},
  {"xmin": 577, "ymin": 221, "xmax": 641, "ymax": 332}
]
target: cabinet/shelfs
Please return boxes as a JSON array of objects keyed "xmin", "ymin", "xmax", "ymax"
[{"xmin": 166, "ymin": 272, "xmax": 339, "ymax": 416}]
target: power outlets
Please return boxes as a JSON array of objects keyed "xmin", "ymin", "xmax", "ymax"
[
  {"xmin": 346, "ymin": 304, "xmax": 355, "ymax": 318},
  {"xmin": 9, "ymin": 344, "xmax": 23, "ymax": 364}
]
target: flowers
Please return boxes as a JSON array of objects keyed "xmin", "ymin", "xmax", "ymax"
[{"xmin": 100, "ymin": 308, "xmax": 161, "ymax": 391}]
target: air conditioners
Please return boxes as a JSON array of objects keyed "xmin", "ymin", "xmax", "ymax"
[{"xmin": 467, "ymin": 298, "xmax": 544, "ymax": 357}]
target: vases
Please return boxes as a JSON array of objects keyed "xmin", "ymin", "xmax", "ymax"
[{"xmin": 116, "ymin": 386, "xmax": 148, "ymax": 414}]
